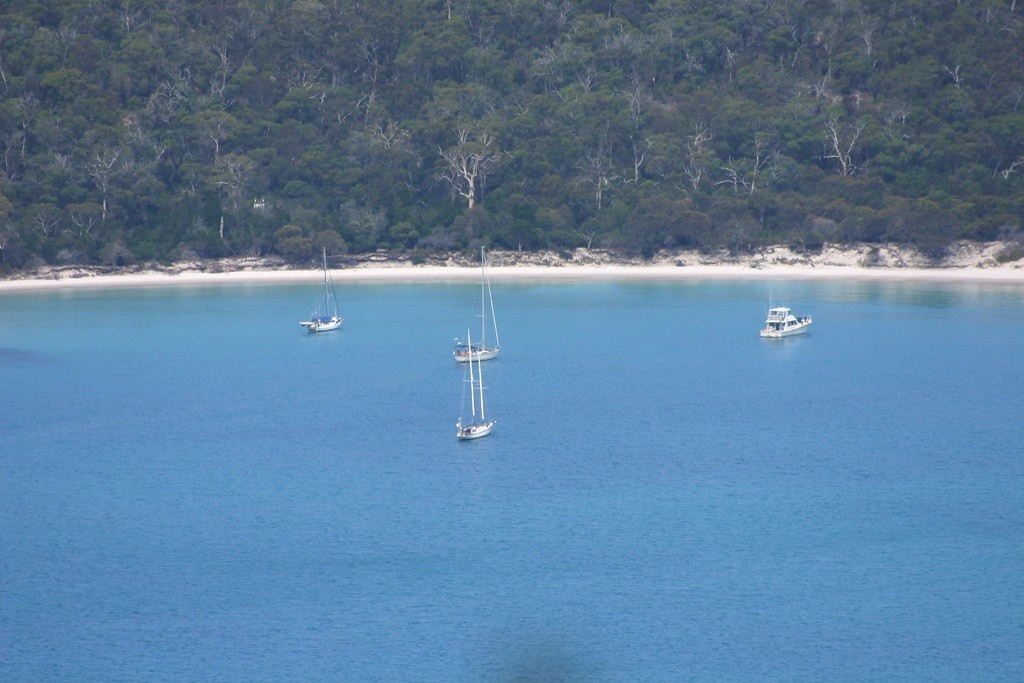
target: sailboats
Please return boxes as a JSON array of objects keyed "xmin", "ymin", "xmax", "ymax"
[
  {"xmin": 451, "ymin": 326, "xmax": 499, "ymax": 440},
  {"xmin": 447, "ymin": 246, "xmax": 503, "ymax": 364},
  {"xmin": 299, "ymin": 246, "xmax": 345, "ymax": 334}
]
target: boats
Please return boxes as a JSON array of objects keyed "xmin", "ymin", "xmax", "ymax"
[{"xmin": 757, "ymin": 288, "xmax": 814, "ymax": 339}]
